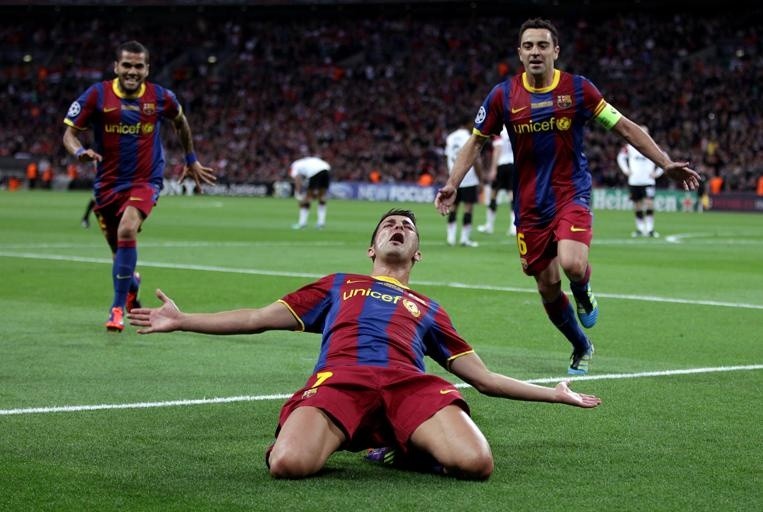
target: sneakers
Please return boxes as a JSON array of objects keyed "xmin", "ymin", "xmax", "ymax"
[
  {"xmin": 631, "ymin": 229, "xmax": 662, "ymax": 239},
  {"xmin": 445, "ymin": 238, "xmax": 480, "ymax": 247},
  {"xmin": 476, "ymin": 223, "xmax": 495, "ymax": 234},
  {"xmin": 317, "ymin": 223, "xmax": 325, "ymax": 231},
  {"xmin": 105, "ymin": 305, "xmax": 127, "ymax": 332},
  {"xmin": 570, "ymin": 274, "xmax": 600, "ymax": 328},
  {"xmin": 291, "ymin": 223, "xmax": 311, "ymax": 231},
  {"xmin": 360, "ymin": 446, "xmax": 401, "ymax": 469},
  {"xmin": 126, "ymin": 273, "xmax": 141, "ymax": 314},
  {"xmin": 568, "ymin": 341, "xmax": 595, "ymax": 378}
]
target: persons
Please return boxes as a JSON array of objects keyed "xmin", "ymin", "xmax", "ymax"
[
  {"xmin": 126, "ymin": 209, "xmax": 602, "ymax": 481},
  {"xmin": 434, "ymin": 20, "xmax": 702, "ymax": 377},
  {"xmin": 61, "ymin": 41, "xmax": 217, "ymax": 330},
  {"xmin": 616, "ymin": 125, "xmax": 668, "ymax": 237},
  {"xmin": 443, "ymin": 116, "xmax": 479, "ymax": 247},
  {"xmin": 476, "ymin": 123, "xmax": 517, "ymax": 236},
  {"xmin": 287, "ymin": 156, "xmax": 331, "ymax": 230}
]
